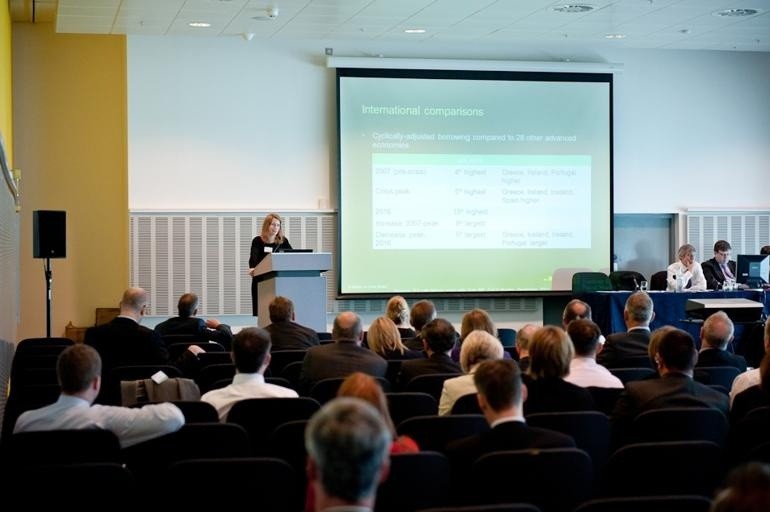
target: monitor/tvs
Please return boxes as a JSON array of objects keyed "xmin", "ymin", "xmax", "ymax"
[
  {"xmin": 284, "ymin": 249, "xmax": 312, "ymax": 252},
  {"xmin": 736, "ymin": 254, "xmax": 770, "ymax": 291}
]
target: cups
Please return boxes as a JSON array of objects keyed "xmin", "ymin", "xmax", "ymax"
[
  {"xmin": 724, "ymin": 280, "xmax": 732, "ymax": 290},
  {"xmin": 641, "ymin": 281, "xmax": 648, "ymax": 291},
  {"xmin": 676, "ymin": 279, "xmax": 684, "ymax": 292}
]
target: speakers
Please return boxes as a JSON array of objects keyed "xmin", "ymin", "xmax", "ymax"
[{"xmin": 33, "ymin": 210, "xmax": 66, "ymax": 259}]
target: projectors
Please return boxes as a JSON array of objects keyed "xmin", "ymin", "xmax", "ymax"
[{"xmin": 684, "ymin": 297, "xmax": 764, "ymax": 323}]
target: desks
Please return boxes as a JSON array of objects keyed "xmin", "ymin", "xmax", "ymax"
[{"xmin": 576, "ymin": 287, "xmax": 767, "ymax": 349}]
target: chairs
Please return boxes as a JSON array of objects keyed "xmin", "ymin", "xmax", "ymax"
[{"xmin": 574, "ymin": 268, "xmax": 668, "ymax": 293}]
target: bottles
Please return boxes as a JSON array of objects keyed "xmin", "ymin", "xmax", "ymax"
[{"xmin": 14, "ymin": 169, "xmax": 22, "ymax": 212}]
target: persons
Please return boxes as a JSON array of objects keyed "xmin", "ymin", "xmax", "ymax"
[
  {"xmin": 760, "ymin": 245, "xmax": 770, "ymax": 255},
  {"xmin": 664, "ymin": 244, "xmax": 708, "ymax": 291},
  {"xmin": 246, "ymin": 213, "xmax": 294, "ymax": 317},
  {"xmin": 702, "ymin": 239, "xmax": 736, "ymax": 291},
  {"xmin": 1, "ymin": 287, "xmax": 769, "ymax": 512}
]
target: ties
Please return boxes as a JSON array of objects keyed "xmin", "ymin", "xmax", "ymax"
[
  {"xmin": 686, "ymin": 269, "xmax": 691, "ymax": 288},
  {"xmin": 722, "ymin": 265, "xmax": 733, "ymax": 278}
]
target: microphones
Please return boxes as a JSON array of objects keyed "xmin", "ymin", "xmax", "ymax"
[
  {"xmin": 708, "ymin": 273, "xmax": 723, "ymax": 287},
  {"xmin": 274, "ymin": 240, "xmax": 283, "ymax": 253},
  {"xmin": 631, "ymin": 274, "xmax": 639, "ymax": 289}
]
